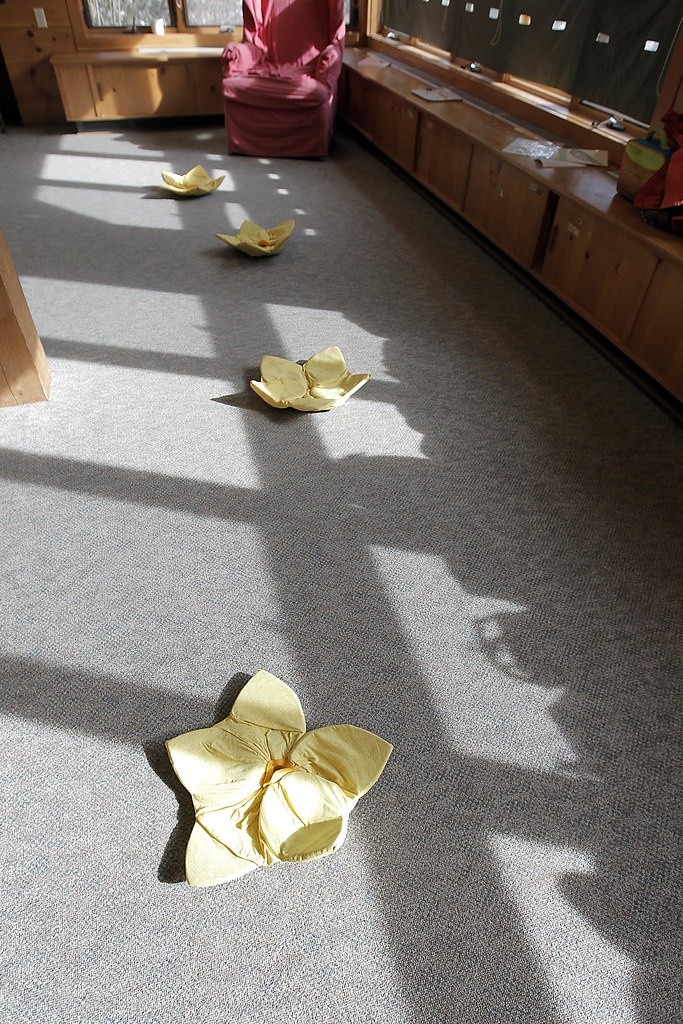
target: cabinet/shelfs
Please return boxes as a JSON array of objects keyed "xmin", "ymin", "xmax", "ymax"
[
  {"xmin": 50, "ymin": 48, "xmax": 228, "ymax": 131},
  {"xmin": 337, "ymin": 46, "xmax": 683, "ymax": 427}
]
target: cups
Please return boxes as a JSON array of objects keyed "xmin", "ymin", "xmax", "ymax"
[{"xmin": 150, "ymin": 17, "xmax": 165, "ymax": 36}]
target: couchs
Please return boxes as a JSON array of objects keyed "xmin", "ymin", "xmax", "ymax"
[{"xmin": 221, "ymin": 0, "xmax": 345, "ymax": 158}]
